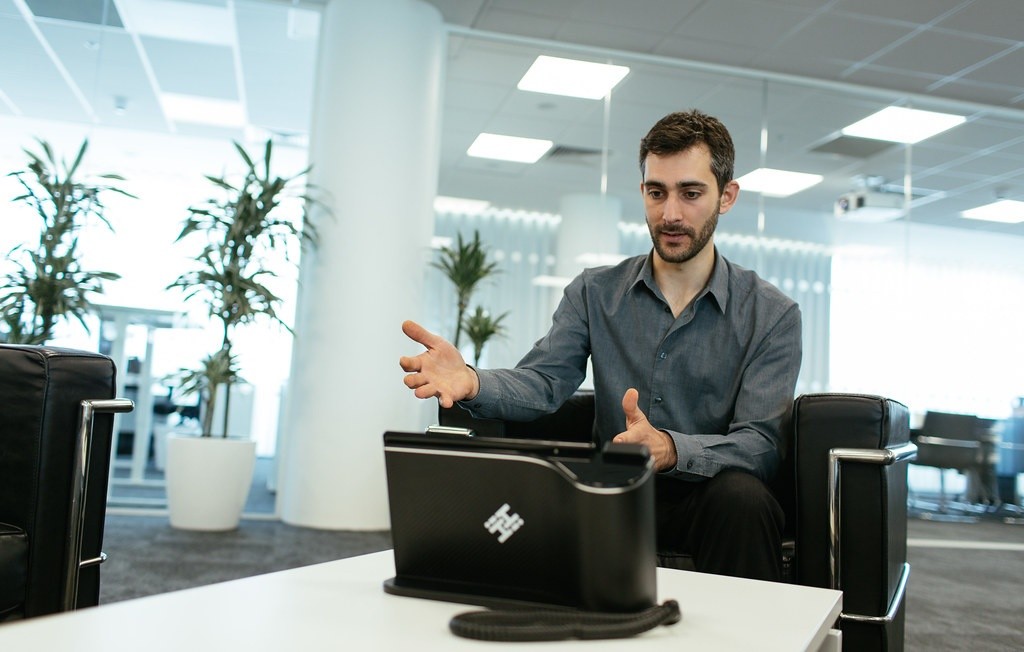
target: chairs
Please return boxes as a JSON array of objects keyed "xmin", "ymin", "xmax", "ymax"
[{"xmin": 905, "ymin": 409, "xmax": 999, "ymax": 521}]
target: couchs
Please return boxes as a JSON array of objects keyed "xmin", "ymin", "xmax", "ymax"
[{"xmin": 434, "ymin": 375, "xmax": 916, "ymax": 652}]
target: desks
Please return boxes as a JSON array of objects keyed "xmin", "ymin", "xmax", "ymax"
[{"xmin": 1, "ymin": 517, "xmax": 858, "ymax": 652}]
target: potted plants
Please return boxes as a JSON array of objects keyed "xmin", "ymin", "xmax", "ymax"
[{"xmin": 158, "ymin": 135, "xmax": 316, "ymax": 535}]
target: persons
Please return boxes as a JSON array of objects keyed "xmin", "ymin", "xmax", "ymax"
[{"xmin": 399, "ymin": 108, "xmax": 803, "ymax": 585}]
